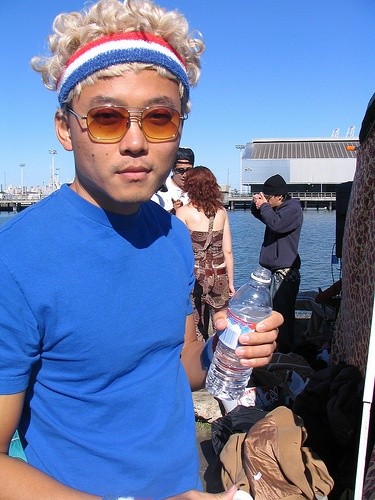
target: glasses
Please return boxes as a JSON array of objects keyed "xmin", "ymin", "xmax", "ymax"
[
  {"xmin": 173, "ymin": 167, "xmax": 192, "ymax": 175},
  {"xmin": 66, "ymin": 103, "xmax": 189, "ymax": 144}
]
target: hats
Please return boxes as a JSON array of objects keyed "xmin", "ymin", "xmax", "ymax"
[
  {"xmin": 263, "ymin": 174, "xmax": 289, "ymax": 195},
  {"xmin": 174, "ymin": 147, "xmax": 194, "ymax": 165}
]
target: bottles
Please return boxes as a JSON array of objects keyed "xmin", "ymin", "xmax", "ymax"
[{"xmin": 204, "ymin": 266, "xmax": 272, "ymax": 402}]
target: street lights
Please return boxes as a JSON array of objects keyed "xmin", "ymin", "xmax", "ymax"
[
  {"xmin": 18, "ymin": 161, "xmax": 26, "ymax": 194},
  {"xmin": 48, "ymin": 148, "xmax": 57, "ymax": 193}
]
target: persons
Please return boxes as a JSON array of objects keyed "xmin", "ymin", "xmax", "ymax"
[
  {"xmin": 150, "ymin": 147, "xmax": 195, "ymax": 213},
  {"xmin": 250, "ymin": 173, "xmax": 303, "ymax": 353},
  {"xmin": 0, "ymin": 0, "xmax": 282, "ymax": 500},
  {"xmin": 176, "ymin": 165, "xmax": 234, "ymax": 337}
]
